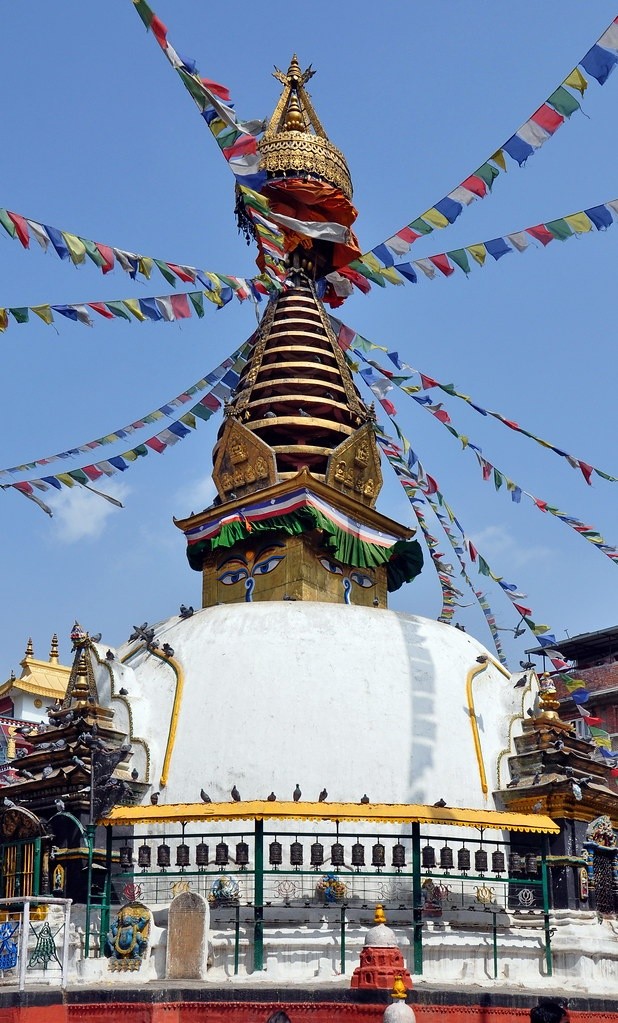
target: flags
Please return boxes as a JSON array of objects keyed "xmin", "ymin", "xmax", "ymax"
[{"xmin": 0, "ymin": 1, "xmax": 618, "ymax": 788}]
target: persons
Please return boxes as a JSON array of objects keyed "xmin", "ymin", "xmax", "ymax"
[
  {"xmin": 213, "ymin": 877, "xmax": 237, "ymax": 898},
  {"xmin": 530, "ymin": 1003, "xmax": 570, "ymax": 1023},
  {"xmin": 113, "ymin": 907, "xmax": 150, "ymax": 959},
  {"xmin": 455, "ymin": 622, "xmax": 461, "ymax": 628},
  {"xmin": 317, "ymin": 873, "xmax": 345, "ymax": 902},
  {"xmin": 421, "ymin": 878, "xmax": 442, "ymax": 909}
]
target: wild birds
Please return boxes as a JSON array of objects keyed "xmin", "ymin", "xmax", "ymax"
[
  {"xmin": 361, "ymin": 794, "xmax": 369, "ymax": 803},
  {"xmin": 514, "ymin": 660, "xmax": 536, "ymax": 688},
  {"xmin": 200, "ymin": 785, "xmax": 240, "ymax": 802},
  {"xmin": 435, "ymin": 798, "xmax": 446, "ymax": 807},
  {"xmin": 325, "ymin": 393, "xmax": 335, "ymax": 400},
  {"xmin": 319, "ymin": 788, "xmax": 328, "ymax": 802},
  {"xmin": 131, "ymin": 768, "xmax": 160, "ymax": 804},
  {"xmin": 299, "ymin": 408, "xmax": 311, "ymax": 417},
  {"xmin": 283, "ymin": 593, "xmax": 297, "ymax": 601},
  {"xmin": 372, "ymin": 597, "xmax": 379, "ymax": 607},
  {"xmin": 313, "ymin": 354, "xmax": 322, "ymax": 363},
  {"xmin": 88, "ymin": 604, "xmax": 194, "ymax": 695},
  {"xmin": 267, "ymin": 784, "xmax": 301, "ymax": 801},
  {"xmin": 476, "ymin": 655, "xmax": 488, "ymax": 663},
  {"xmin": 264, "ymin": 412, "xmax": 277, "ymax": 418},
  {"xmin": 566, "ymin": 766, "xmax": 592, "ymax": 784},
  {"xmin": 507, "ymin": 772, "xmax": 542, "ymax": 788},
  {"xmin": 455, "ymin": 622, "xmax": 465, "ymax": 632},
  {"xmin": 532, "ymin": 800, "xmax": 542, "ymax": 814},
  {"xmin": 527, "ymin": 708, "xmax": 533, "ymax": 716}
]
